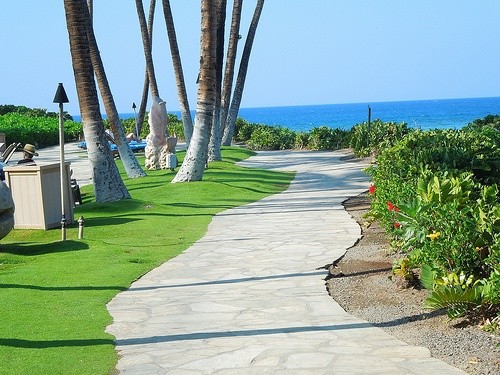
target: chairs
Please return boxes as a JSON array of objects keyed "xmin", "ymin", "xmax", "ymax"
[{"xmin": 77, "ymin": 131, "xmax": 148, "ymax": 156}]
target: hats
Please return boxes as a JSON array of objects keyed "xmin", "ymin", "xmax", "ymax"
[{"xmin": 22, "ymin": 144, "xmax": 38, "ymax": 156}]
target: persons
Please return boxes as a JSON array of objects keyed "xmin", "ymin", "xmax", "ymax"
[
  {"xmin": 16, "ymin": 144, "xmax": 39, "ymax": 165},
  {"xmin": 106, "ymin": 133, "xmax": 142, "ymax": 145}
]
